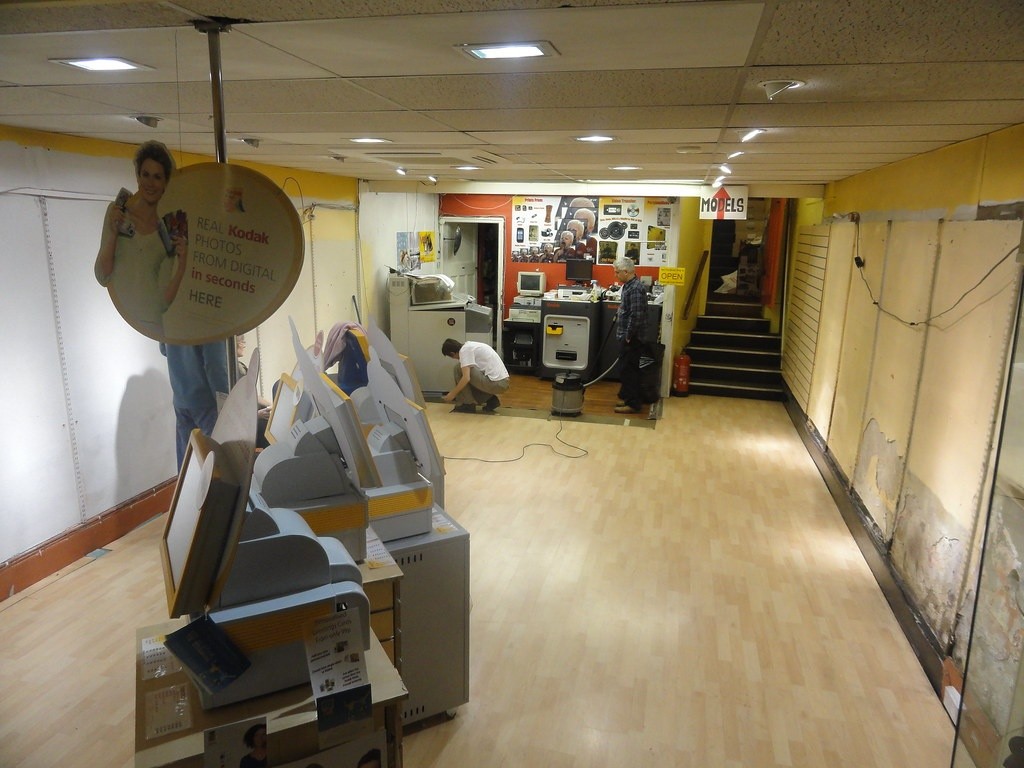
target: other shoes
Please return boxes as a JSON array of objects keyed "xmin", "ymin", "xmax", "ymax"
[
  {"xmin": 614, "ymin": 406, "xmax": 641, "ymax": 413},
  {"xmin": 616, "ymin": 402, "xmax": 625, "ymax": 406}
]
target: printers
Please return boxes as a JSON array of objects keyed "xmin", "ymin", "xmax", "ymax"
[{"xmin": 389, "ymin": 276, "xmax": 493, "ymax": 403}]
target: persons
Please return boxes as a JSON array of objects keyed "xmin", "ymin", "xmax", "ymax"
[
  {"xmin": 512, "ymin": 197, "xmax": 597, "ymax": 265},
  {"xmin": 161, "ymin": 330, "xmax": 276, "ymax": 476},
  {"xmin": 600, "ymin": 243, "xmax": 613, "ymax": 259},
  {"xmin": 239, "ymin": 724, "xmax": 267, "ymax": 768},
  {"xmin": 613, "ymin": 256, "xmax": 649, "ymax": 413},
  {"xmin": 94, "ymin": 140, "xmax": 188, "ymax": 341},
  {"xmin": 626, "ymin": 244, "xmax": 639, "ymax": 265},
  {"xmin": 482, "ymin": 226, "xmax": 496, "ymax": 305},
  {"xmin": 441, "ymin": 338, "xmax": 509, "ymax": 413}
]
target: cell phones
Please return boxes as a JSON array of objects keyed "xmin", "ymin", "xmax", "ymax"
[{"xmin": 517, "ymin": 228, "xmax": 524, "ymax": 243}]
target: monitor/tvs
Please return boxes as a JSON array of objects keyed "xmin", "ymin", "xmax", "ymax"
[
  {"xmin": 264, "ymin": 352, "xmax": 446, "ymax": 489},
  {"xmin": 517, "ymin": 272, "xmax": 546, "ymax": 297},
  {"xmin": 566, "ymin": 260, "xmax": 593, "ymax": 286},
  {"xmin": 160, "ymin": 428, "xmax": 243, "ymax": 620}
]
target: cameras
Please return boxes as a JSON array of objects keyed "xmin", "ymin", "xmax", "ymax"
[{"xmin": 117, "ymin": 218, "xmax": 135, "ymax": 238}]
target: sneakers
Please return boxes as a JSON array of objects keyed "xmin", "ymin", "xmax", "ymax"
[
  {"xmin": 455, "ymin": 404, "xmax": 477, "ymax": 413},
  {"xmin": 482, "ymin": 398, "xmax": 501, "ymax": 412}
]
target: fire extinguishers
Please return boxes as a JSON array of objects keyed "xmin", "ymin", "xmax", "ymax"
[{"xmin": 673, "ymin": 346, "xmax": 690, "ymax": 397}]
